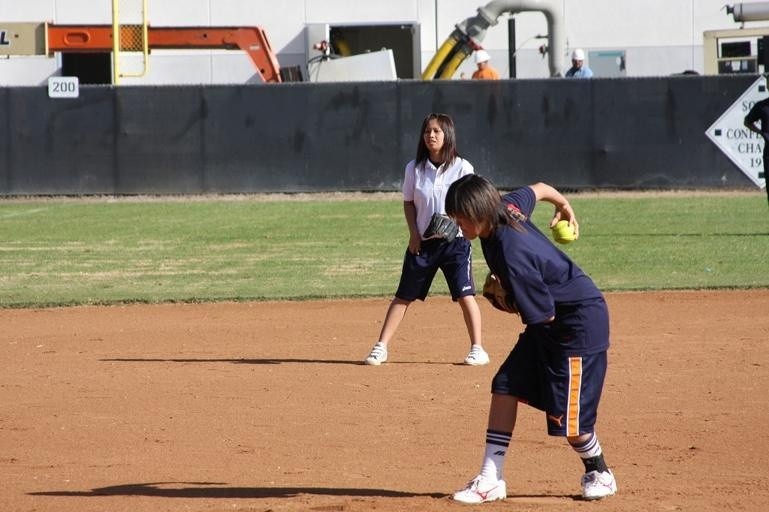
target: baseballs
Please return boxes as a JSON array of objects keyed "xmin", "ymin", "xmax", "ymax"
[{"xmin": 552, "ymin": 220, "xmax": 575, "ymax": 243}]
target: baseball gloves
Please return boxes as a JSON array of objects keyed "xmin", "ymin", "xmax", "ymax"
[
  {"xmin": 483, "ymin": 270, "xmax": 518, "ymax": 313},
  {"xmin": 423, "ymin": 212, "xmax": 458, "ymax": 241}
]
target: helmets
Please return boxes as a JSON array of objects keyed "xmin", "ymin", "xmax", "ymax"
[
  {"xmin": 572, "ymin": 49, "xmax": 584, "ymax": 60},
  {"xmin": 475, "ymin": 50, "xmax": 489, "ymax": 63}
]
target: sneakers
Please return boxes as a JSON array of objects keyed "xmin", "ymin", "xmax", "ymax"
[
  {"xmin": 580, "ymin": 467, "xmax": 617, "ymax": 500},
  {"xmin": 464, "ymin": 344, "xmax": 490, "ymax": 366},
  {"xmin": 365, "ymin": 341, "xmax": 388, "ymax": 366},
  {"xmin": 452, "ymin": 475, "xmax": 507, "ymax": 503}
]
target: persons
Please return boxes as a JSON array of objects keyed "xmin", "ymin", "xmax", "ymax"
[
  {"xmin": 363, "ymin": 112, "xmax": 492, "ymax": 368},
  {"xmin": 444, "ymin": 171, "xmax": 619, "ymax": 506},
  {"xmin": 470, "ymin": 48, "xmax": 501, "ymax": 79},
  {"xmin": 565, "ymin": 45, "xmax": 595, "ymax": 78}
]
